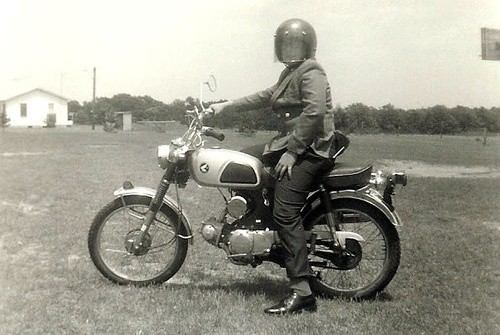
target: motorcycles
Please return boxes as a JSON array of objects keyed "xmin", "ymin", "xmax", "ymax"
[{"xmin": 87, "ymin": 74, "xmax": 407, "ymax": 302}]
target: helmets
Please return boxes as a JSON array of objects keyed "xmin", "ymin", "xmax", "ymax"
[{"xmin": 275, "ymin": 18, "xmax": 316, "ymax": 66}]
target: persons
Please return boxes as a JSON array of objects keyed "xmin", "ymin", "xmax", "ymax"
[{"xmin": 209, "ymin": 18, "xmax": 350, "ymax": 316}]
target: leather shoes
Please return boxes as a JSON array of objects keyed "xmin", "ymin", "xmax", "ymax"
[{"xmin": 264, "ymin": 292, "xmax": 317, "ymax": 314}]
